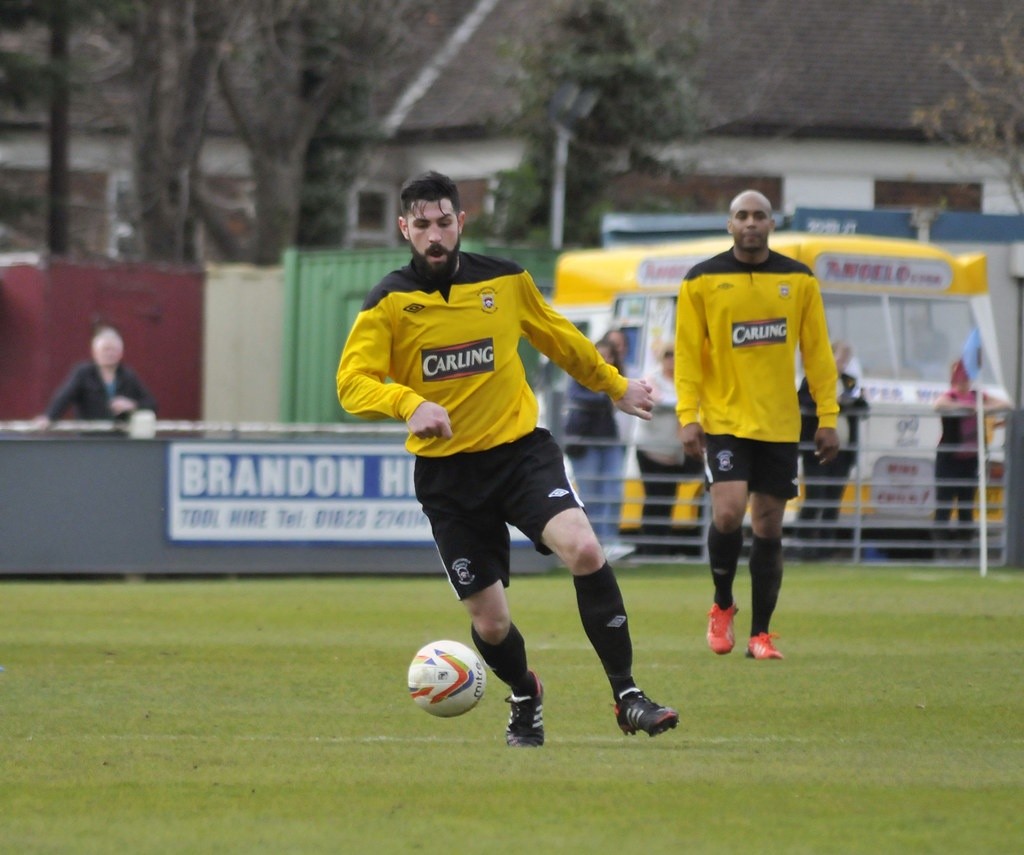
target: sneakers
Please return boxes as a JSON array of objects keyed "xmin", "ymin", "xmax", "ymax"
[
  {"xmin": 746, "ymin": 632, "xmax": 784, "ymax": 659},
  {"xmin": 707, "ymin": 602, "xmax": 737, "ymax": 655},
  {"xmin": 614, "ymin": 692, "xmax": 679, "ymax": 736},
  {"xmin": 505, "ymin": 670, "xmax": 545, "ymax": 748}
]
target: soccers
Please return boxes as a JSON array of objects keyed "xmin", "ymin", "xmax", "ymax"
[{"xmin": 407, "ymin": 638, "xmax": 486, "ymax": 718}]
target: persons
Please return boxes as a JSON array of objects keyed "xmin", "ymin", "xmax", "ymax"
[
  {"xmin": 31, "ymin": 322, "xmax": 157, "ymax": 431},
  {"xmin": 562, "ymin": 330, "xmax": 704, "ymax": 563},
  {"xmin": 673, "ymin": 189, "xmax": 841, "ymax": 661},
  {"xmin": 934, "ymin": 361, "xmax": 1008, "ymax": 559},
  {"xmin": 336, "ymin": 171, "xmax": 678, "ymax": 748},
  {"xmin": 783, "ymin": 345, "xmax": 869, "ymax": 561}
]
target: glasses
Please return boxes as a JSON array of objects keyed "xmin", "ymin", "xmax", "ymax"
[{"xmin": 664, "ymin": 351, "xmax": 673, "ymax": 358}]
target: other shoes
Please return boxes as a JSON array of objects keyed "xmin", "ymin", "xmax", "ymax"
[{"xmin": 600, "ymin": 544, "xmax": 635, "ymax": 563}]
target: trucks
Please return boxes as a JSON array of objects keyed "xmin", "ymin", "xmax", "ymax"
[{"xmin": 535, "ymin": 230, "xmax": 1011, "ymax": 555}]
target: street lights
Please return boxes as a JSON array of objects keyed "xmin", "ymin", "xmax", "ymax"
[{"xmin": 548, "ymin": 80, "xmax": 604, "ymax": 249}]
